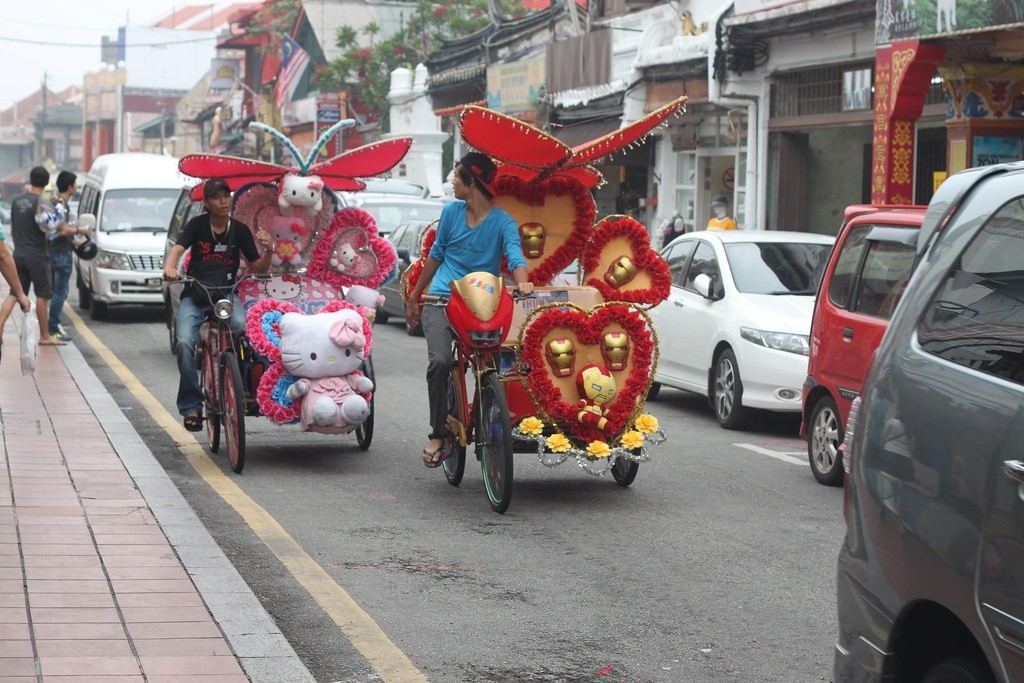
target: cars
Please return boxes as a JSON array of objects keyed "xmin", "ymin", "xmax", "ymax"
[
  {"xmin": 343, "ymin": 191, "xmax": 447, "ymax": 240},
  {"xmin": 633, "ymin": 230, "xmax": 837, "ymax": 431},
  {"xmin": 375, "ymin": 221, "xmax": 435, "ymax": 339}
]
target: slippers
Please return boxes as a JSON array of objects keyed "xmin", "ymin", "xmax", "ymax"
[
  {"xmin": 39, "ymin": 338, "xmax": 67, "ymax": 345},
  {"xmin": 183, "ymin": 410, "xmax": 203, "ymax": 432},
  {"xmin": 489, "ymin": 406, "xmax": 517, "ymax": 421},
  {"xmin": 422, "ymin": 436, "xmax": 445, "ymax": 468}
]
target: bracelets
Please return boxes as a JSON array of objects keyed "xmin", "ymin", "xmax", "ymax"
[{"xmin": 75, "ymin": 226, "xmax": 79, "ymax": 235}]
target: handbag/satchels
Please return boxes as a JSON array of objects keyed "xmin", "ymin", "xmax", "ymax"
[{"xmin": 19, "ymin": 297, "xmax": 41, "ymax": 376}]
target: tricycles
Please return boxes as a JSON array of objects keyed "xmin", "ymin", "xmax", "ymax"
[
  {"xmin": 419, "ymin": 294, "xmax": 641, "ymax": 517},
  {"xmin": 162, "ymin": 272, "xmax": 375, "ymax": 475}
]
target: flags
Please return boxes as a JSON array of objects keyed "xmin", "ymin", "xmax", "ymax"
[{"xmin": 278, "ymin": 34, "xmax": 311, "ymax": 109}]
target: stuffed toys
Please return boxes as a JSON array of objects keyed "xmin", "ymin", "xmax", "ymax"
[
  {"xmin": 272, "ymin": 171, "xmax": 361, "ymax": 272},
  {"xmin": 283, "ymin": 309, "xmax": 375, "ymax": 433}
]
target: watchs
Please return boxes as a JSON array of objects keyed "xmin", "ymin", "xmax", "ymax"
[{"xmin": 265, "ymin": 244, "xmax": 276, "ymax": 253}]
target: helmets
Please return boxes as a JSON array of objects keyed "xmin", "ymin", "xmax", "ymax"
[{"xmin": 72, "ymin": 234, "xmax": 97, "ymax": 260}]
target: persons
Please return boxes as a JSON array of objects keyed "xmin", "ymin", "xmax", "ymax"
[
  {"xmin": 662, "ymin": 195, "xmax": 737, "ymax": 249},
  {"xmin": 0, "ymin": 166, "xmax": 93, "ymax": 364},
  {"xmin": 407, "ymin": 153, "xmax": 536, "ymax": 468},
  {"xmin": 162, "ymin": 179, "xmax": 274, "ymax": 431}
]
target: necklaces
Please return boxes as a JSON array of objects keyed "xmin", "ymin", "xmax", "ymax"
[{"xmin": 209, "ymin": 212, "xmax": 231, "ymax": 243}]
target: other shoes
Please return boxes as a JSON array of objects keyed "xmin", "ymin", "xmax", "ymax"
[{"xmin": 49, "ymin": 330, "xmax": 72, "ymax": 340}]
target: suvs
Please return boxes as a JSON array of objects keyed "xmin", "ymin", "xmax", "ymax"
[
  {"xmin": 832, "ymin": 158, "xmax": 1024, "ymax": 683},
  {"xmin": 797, "ymin": 202, "xmax": 926, "ymax": 490}
]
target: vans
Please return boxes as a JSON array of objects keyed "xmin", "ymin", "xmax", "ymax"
[
  {"xmin": 162, "ymin": 183, "xmax": 205, "ymax": 302},
  {"xmin": 74, "ymin": 152, "xmax": 200, "ymax": 322}
]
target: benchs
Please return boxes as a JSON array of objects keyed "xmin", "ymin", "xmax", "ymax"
[
  {"xmin": 239, "ymin": 273, "xmax": 347, "ymax": 398},
  {"xmin": 497, "ymin": 286, "xmax": 605, "ymax": 420}
]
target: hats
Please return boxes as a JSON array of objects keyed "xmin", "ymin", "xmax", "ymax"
[
  {"xmin": 204, "ymin": 179, "xmax": 232, "ymax": 197},
  {"xmin": 462, "ymin": 151, "xmax": 497, "ymax": 198},
  {"xmin": 709, "ymin": 195, "xmax": 729, "ymax": 207}
]
